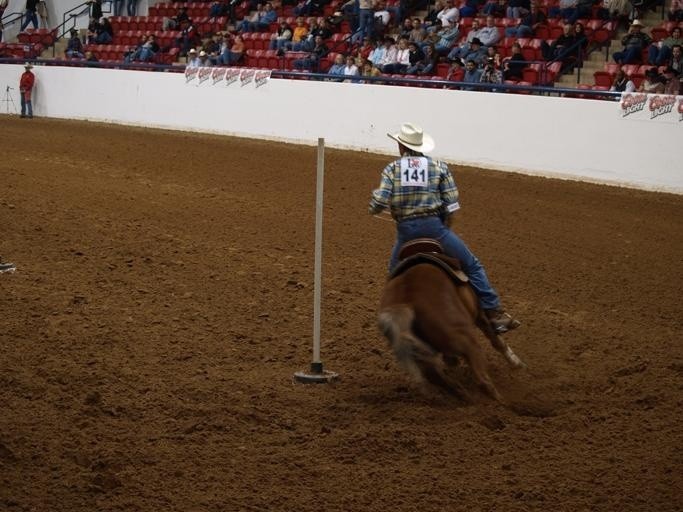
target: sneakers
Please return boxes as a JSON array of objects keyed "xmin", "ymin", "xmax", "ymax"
[
  {"xmin": 19, "ymin": 114, "xmax": 33, "ymax": 117},
  {"xmin": 20, "ymin": 28, "xmax": 39, "ymax": 34},
  {"xmin": 489, "ymin": 309, "xmax": 521, "ymax": 333}
]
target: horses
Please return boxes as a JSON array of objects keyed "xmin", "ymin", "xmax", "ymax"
[{"xmin": 371, "ymin": 262, "xmax": 529, "ymax": 428}]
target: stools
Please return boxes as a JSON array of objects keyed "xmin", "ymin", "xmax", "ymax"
[
  {"xmin": 0, "ymin": 26, "xmax": 59, "ymax": 64},
  {"xmin": 79, "ymin": 0, "xmax": 566, "ymax": 98}
]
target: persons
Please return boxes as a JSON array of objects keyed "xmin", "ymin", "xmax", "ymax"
[
  {"xmin": 367, "ymin": 123, "xmax": 522, "ymax": 334},
  {"xmin": 0, "ymin": 0, "xmax": 683, "ymax": 96},
  {"xmin": 19, "ymin": 61, "xmax": 34, "ymax": 118}
]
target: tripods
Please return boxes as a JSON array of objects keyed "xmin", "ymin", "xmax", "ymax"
[{"xmin": 0, "ymin": 91, "xmax": 20, "ymax": 117}]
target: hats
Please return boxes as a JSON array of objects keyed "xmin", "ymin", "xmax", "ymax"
[
  {"xmin": 187, "ymin": 48, "xmax": 199, "ymax": 56},
  {"xmin": 199, "ymin": 50, "xmax": 207, "ymax": 58},
  {"xmin": 23, "ymin": 61, "xmax": 33, "ymax": 69},
  {"xmin": 663, "ymin": 66, "xmax": 680, "ymax": 77},
  {"xmin": 446, "ymin": 15, "xmax": 458, "ymax": 23},
  {"xmin": 448, "ymin": 57, "xmax": 465, "ymax": 67},
  {"xmin": 630, "ymin": 19, "xmax": 646, "ymax": 28},
  {"xmin": 387, "ymin": 122, "xmax": 435, "ymax": 153},
  {"xmin": 280, "ymin": 20, "xmax": 286, "ymax": 25},
  {"xmin": 469, "ymin": 38, "xmax": 484, "ymax": 46},
  {"xmin": 645, "ymin": 68, "xmax": 662, "ymax": 77},
  {"xmin": 70, "ymin": 29, "xmax": 78, "ymax": 36},
  {"xmin": 374, "ymin": 35, "xmax": 395, "ymax": 43}
]
